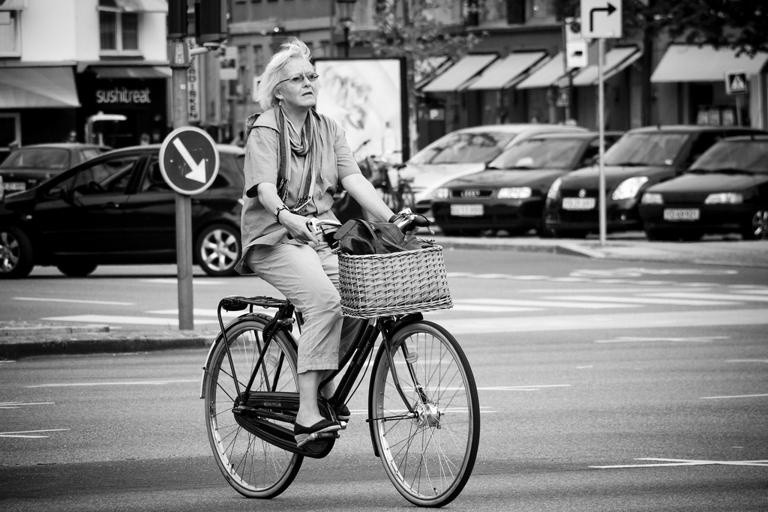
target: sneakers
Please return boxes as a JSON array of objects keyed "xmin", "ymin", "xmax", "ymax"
[
  {"xmin": 338, "ymin": 403, "xmax": 351, "ymax": 419},
  {"xmin": 294, "ymin": 419, "xmax": 341, "ymax": 447}
]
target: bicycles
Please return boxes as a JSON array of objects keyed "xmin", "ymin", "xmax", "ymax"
[
  {"xmin": 200, "ymin": 207, "xmax": 481, "ymax": 508},
  {"xmin": 352, "ymin": 139, "xmax": 415, "ymax": 235}
]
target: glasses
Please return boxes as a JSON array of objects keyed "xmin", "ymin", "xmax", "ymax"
[{"xmin": 276, "ymin": 72, "xmax": 319, "ymax": 88}]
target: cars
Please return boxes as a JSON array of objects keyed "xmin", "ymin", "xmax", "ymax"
[
  {"xmin": 0, "ymin": 142, "xmax": 246, "ymax": 279},
  {"xmin": 430, "ymin": 131, "xmax": 663, "ymax": 237},
  {"xmin": 395, "ymin": 122, "xmax": 592, "ymax": 217},
  {"xmin": 542, "ymin": 125, "xmax": 768, "ymax": 238},
  {"xmin": 639, "ymin": 134, "xmax": 768, "ymax": 242}
]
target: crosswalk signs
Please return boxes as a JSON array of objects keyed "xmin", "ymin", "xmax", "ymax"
[{"xmin": 728, "ymin": 73, "xmax": 747, "ymax": 94}]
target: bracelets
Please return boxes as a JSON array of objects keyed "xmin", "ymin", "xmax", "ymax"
[
  {"xmin": 386, "ymin": 213, "xmax": 399, "ymax": 225},
  {"xmin": 272, "ymin": 204, "xmax": 290, "ymax": 227}
]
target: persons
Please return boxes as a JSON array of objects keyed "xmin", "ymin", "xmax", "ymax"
[{"xmin": 233, "ymin": 36, "xmax": 437, "ymax": 452}]
